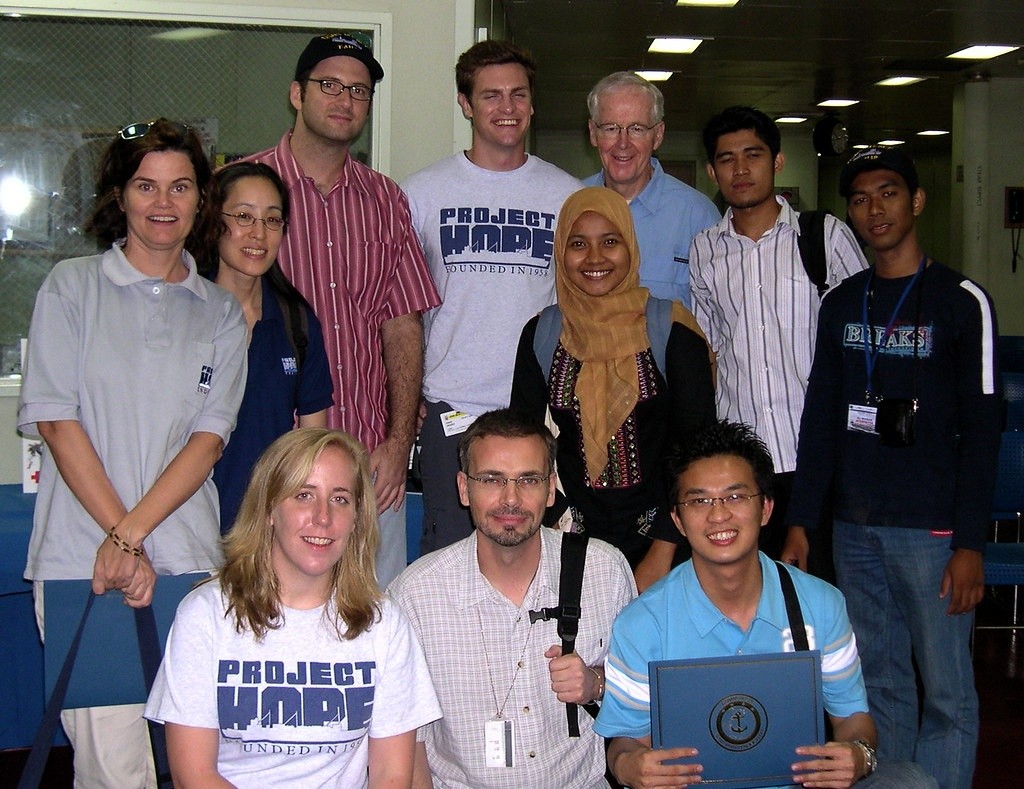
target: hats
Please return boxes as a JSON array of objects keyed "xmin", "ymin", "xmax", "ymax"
[
  {"xmin": 838, "ymin": 147, "xmax": 919, "ymax": 196},
  {"xmin": 294, "ymin": 31, "xmax": 384, "ymax": 102}
]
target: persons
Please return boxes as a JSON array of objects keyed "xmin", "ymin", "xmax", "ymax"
[
  {"xmin": 583, "ymin": 71, "xmax": 722, "ymax": 312},
  {"xmin": 211, "ymin": 29, "xmax": 443, "ymax": 592},
  {"xmin": 593, "ymin": 420, "xmax": 940, "ymax": 789},
  {"xmin": 382, "ymin": 409, "xmax": 639, "ymax": 789},
  {"xmin": 184, "ymin": 163, "xmax": 336, "ymax": 536},
  {"xmin": 510, "ymin": 186, "xmax": 719, "ymax": 595},
  {"xmin": 781, "ymin": 132, "xmax": 1001, "ymax": 789},
  {"xmin": 18, "ymin": 119, "xmax": 248, "ymax": 789},
  {"xmin": 688, "ymin": 106, "xmax": 871, "ymax": 589},
  {"xmin": 144, "ymin": 427, "xmax": 444, "ymax": 789},
  {"xmin": 397, "ymin": 40, "xmax": 588, "ymax": 557}
]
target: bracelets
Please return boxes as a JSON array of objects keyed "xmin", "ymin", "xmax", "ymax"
[
  {"xmin": 109, "ymin": 526, "xmax": 143, "ymax": 556},
  {"xmin": 588, "ymin": 668, "xmax": 604, "ymax": 701}
]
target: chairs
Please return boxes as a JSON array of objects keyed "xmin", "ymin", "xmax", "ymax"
[{"xmin": 969, "ymin": 333, "xmax": 1024, "ymax": 678}]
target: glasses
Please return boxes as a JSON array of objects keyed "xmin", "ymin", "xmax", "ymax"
[
  {"xmin": 307, "ymin": 78, "xmax": 376, "ymax": 101},
  {"xmin": 117, "ymin": 117, "xmax": 194, "ymax": 140},
  {"xmin": 466, "ymin": 475, "xmax": 551, "ymax": 490},
  {"xmin": 593, "ymin": 122, "xmax": 662, "ymax": 139},
  {"xmin": 222, "ymin": 212, "xmax": 290, "ymax": 231},
  {"xmin": 677, "ymin": 492, "xmax": 760, "ymax": 512}
]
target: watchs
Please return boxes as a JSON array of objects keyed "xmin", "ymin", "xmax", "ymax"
[{"xmin": 853, "ymin": 739, "xmax": 879, "ymax": 780}]
